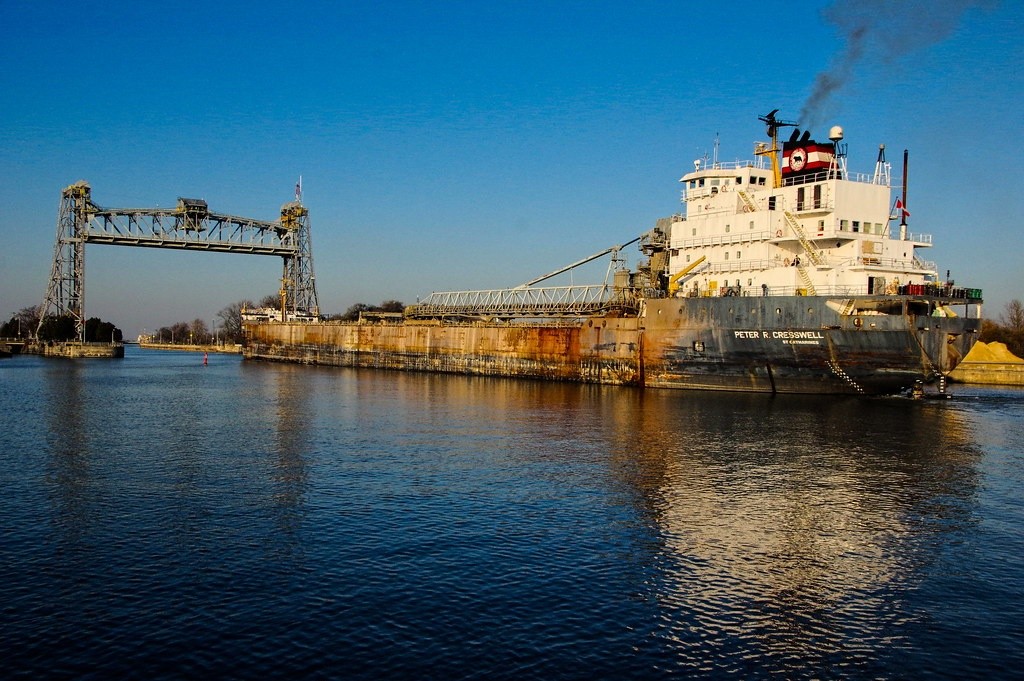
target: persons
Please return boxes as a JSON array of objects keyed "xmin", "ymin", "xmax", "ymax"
[
  {"xmin": 791, "ymin": 254, "xmax": 801, "ymax": 265},
  {"xmin": 203, "ymin": 351, "xmax": 208, "ymax": 364}
]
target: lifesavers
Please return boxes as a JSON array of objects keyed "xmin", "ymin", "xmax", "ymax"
[
  {"xmin": 783, "ymin": 258, "xmax": 790, "ymax": 267},
  {"xmin": 743, "ymin": 205, "xmax": 749, "ymax": 213},
  {"xmin": 720, "ymin": 185, "xmax": 726, "ymax": 193},
  {"xmin": 776, "ymin": 230, "xmax": 783, "ymax": 238}
]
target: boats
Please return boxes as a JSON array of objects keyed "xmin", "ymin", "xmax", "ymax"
[{"xmin": 242, "ymin": 109, "xmax": 983, "ymax": 397}]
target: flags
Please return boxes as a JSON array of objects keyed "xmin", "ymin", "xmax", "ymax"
[{"xmin": 895, "ymin": 199, "xmax": 911, "ymax": 217}]
